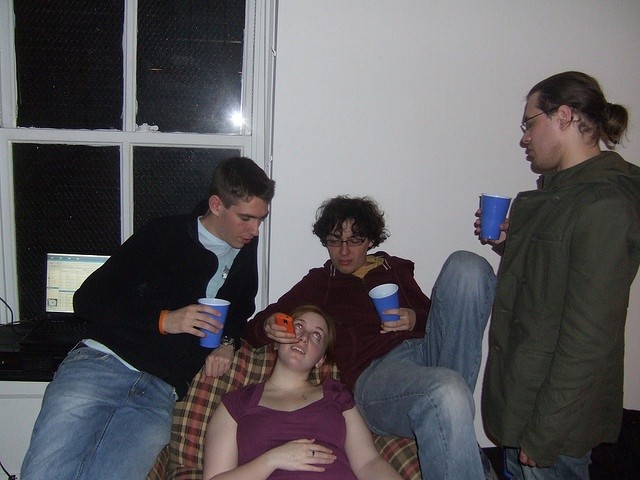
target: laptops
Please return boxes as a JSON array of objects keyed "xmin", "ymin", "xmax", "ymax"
[{"xmin": 19, "ymin": 252, "xmax": 112, "ymax": 350}]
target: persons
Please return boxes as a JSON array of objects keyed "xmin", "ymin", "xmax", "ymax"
[
  {"xmin": 245, "ymin": 197, "xmax": 497, "ymax": 479},
  {"xmin": 476, "ymin": 72, "xmax": 639, "ymax": 478},
  {"xmin": 203, "ymin": 304, "xmax": 403, "ymax": 478},
  {"xmin": 20, "ymin": 156, "xmax": 274, "ymax": 479}
]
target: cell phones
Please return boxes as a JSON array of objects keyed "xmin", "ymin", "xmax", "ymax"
[{"xmin": 276, "ymin": 313, "xmax": 294, "ymax": 334}]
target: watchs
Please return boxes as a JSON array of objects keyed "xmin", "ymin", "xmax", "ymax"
[{"xmin": 221, "ymin": 334, "xmax": 238, "ymax": 347}]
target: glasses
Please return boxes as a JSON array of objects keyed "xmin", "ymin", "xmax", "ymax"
[
  {"xmin": 520, "ymin": 107, "xmax": 560, "ymax": 131},
  {"xmin": 323, "ymin": 236, "xmax": 367, "ymax": 247}
]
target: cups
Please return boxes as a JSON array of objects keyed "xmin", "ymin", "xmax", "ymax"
[
  {"xmin": 479, "ymin": 193, "xmax": 512, "ymax": 242},
  {"xmin": 369, "ymin": 283, "xmax": 403, "ymax": 326},
  {"xmin": 198, "ymin": 298, "xmax": 231, "ymax": 349}
]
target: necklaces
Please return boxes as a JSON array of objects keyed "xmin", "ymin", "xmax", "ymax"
[{"xmin": 268, "ymin": 377, "xmax": 311, "ymax": 401}]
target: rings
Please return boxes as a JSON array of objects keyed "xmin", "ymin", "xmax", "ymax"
[{"xmin": 312, "ymin": 449, "xmax": 315, "ymax": 457}]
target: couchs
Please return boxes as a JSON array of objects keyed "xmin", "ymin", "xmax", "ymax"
[{"xmin": 143, "ymin": 334, "xmax": 423, "ymax": 480}]
